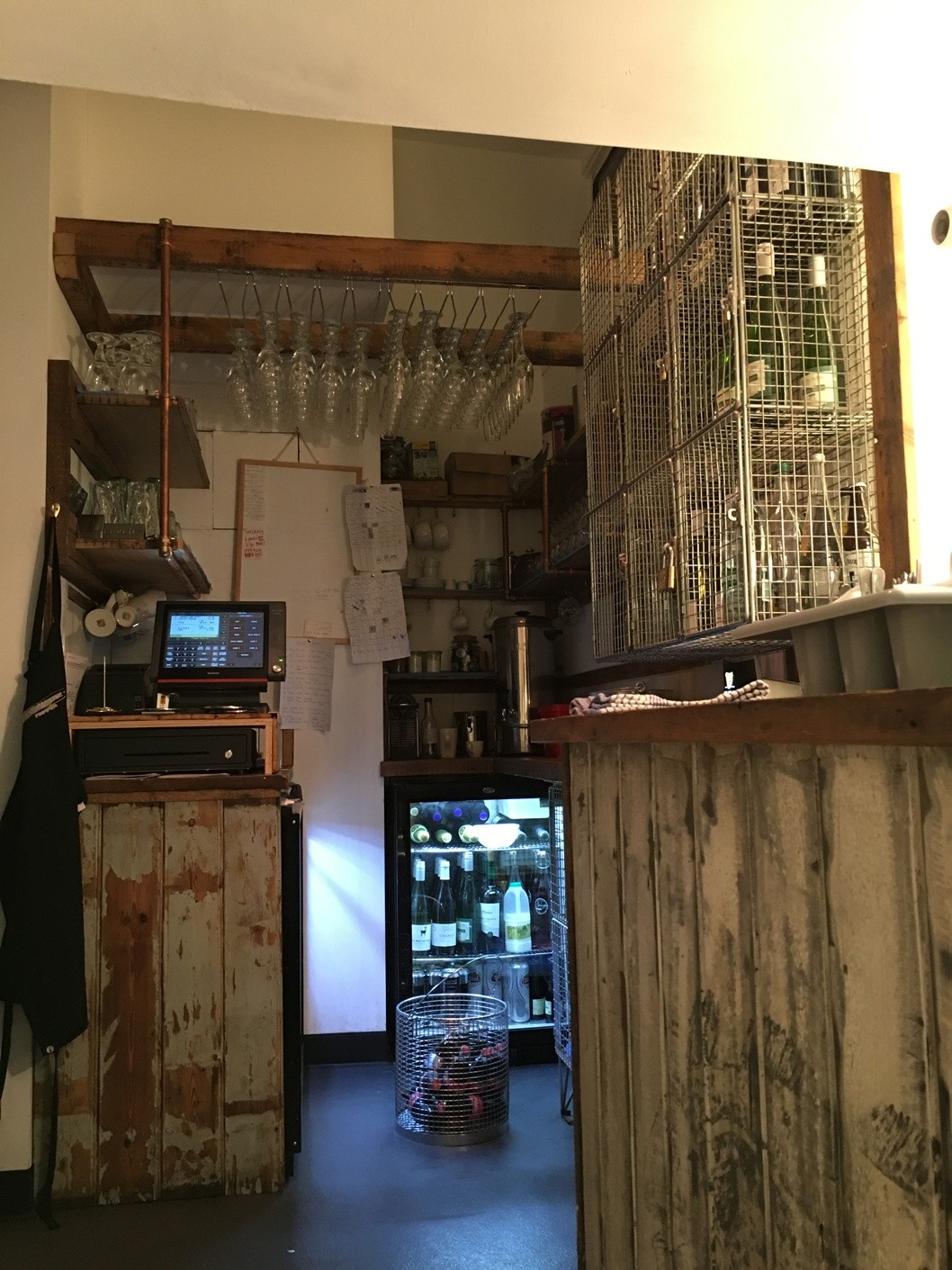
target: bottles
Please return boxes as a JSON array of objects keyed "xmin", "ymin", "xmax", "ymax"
[
  {"xmin": 690, "ymin": 454, "xmax": 881, "ymax": 632},
  {"xmin": 408, "ymin": 800, "xmax": 554, "ymax": 1021},
  {"xmin": 450, "ymin": 634, "xmax": 481, "ymax": 672},
  {"xmin": 714, "ymin": 157, "xmax": 849, "ymax": 436},
  {"xmin": 407, "ymin": 1025, "xmax": 509, "ymax": 1133},
  {"xmin": 474, "ymin": 557, "xmax": 500, "ymax": 588},
  {"xmin": 421, "ymin": 696, "xmax": 440, "ymax": 758}
]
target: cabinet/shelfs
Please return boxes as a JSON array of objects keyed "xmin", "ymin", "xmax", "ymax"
[
  {"xmin": 575, "ymin": 147, "xmax": 883, "ymax": 665},
  {"xmin": 381, "ymin": 495, "xmax": 540, "ymax": 693},
  {"xmin": 41, "ymin": 356, "xmax": 209, "ymax": 608},
  {"xmin": 33, "ymin": 711, "xmax": 302, "ymax": 1207},
  {"xmin": 502, "ymin": 422, "xmax": 590, "ymax": 597}
]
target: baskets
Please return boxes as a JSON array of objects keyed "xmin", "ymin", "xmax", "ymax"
[{"xmin": 393, "ymin": 955, "xmax": 512, "ymax": 1147}]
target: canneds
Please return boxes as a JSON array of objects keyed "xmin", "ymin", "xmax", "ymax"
[{"xmin": 411, "ymin": 957, "xmax": 531, "ymax": 1023}]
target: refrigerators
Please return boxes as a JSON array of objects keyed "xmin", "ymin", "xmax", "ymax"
[{"xmin": 386, "ymin": 773, "xmax": 563, "ymax": 1070}]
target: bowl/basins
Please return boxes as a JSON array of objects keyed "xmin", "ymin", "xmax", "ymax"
[
  {"xmin": 402, "ymin": 576, "xmax": 448, "ymax": 590},
  {"xmin": 473, "ymin": 823, "xmax": 520, "ymax": 849}
]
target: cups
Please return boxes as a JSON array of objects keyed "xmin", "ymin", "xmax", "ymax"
[
  {"xmin": 91, "ymin": 476, "xmax": 182, "ymax": 540},
  {"xmin": 451, "ymin": 607, "xmax": 468, "ymax": 628},
  {"xmin": 484, "ymin": 607, "xmax": 499, "ymax": 628},
  {"xmin": 389, "ymin": 651, "xmax": 442, "ymax": 672},
  {"xmin": 84, "ymin": 593, "xmax": 136, "ymax": 639},
  {"xmin": 440, "ymin": 728, "xmax": 483, "ymax": 759},
  {"xmin": 538, "ymin": 703, "xmax": 571, "ymax": 756},
  {"xmin": 424, "ymin": 565, "xmax": 439, "ymax": 578},
  {"xmin": 405, "ymin": 516, "xmax": 450, "ymax": 552}
]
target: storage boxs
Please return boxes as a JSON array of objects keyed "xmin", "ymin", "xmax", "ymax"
[
  {"xmin": 385, "ymin": 440, "xmax": 448, "ymax": 499},
  {"xmin": 445, "ymin": 452, "xmax": 528, "ymax": 496}
]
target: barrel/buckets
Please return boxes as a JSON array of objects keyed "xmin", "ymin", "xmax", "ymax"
[
  {"xmin": 483, "ymin": 611, "xmax": 567, "ymax": 757},
  {"xmin": 454, "ymin": 711, "xmax": 486, "ymax": 758}
]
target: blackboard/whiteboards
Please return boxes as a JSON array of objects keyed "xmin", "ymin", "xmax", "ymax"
[{"xmin": 232, "ymin": 457, "xmax": 362, "ymax": 645}]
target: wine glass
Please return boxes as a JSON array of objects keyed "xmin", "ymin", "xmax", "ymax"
[
  {"xmin": 82, "ymin": 331, "xmax": 164, "ymax": 392},
  {"xmin": 225, "ymin": 310, "xmax": 535, "ymax": 442}
]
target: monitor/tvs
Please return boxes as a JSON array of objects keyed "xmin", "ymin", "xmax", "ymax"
[{"xmin": 149, "ymin": 599, "xmax": 288, "ymax": 709}]
